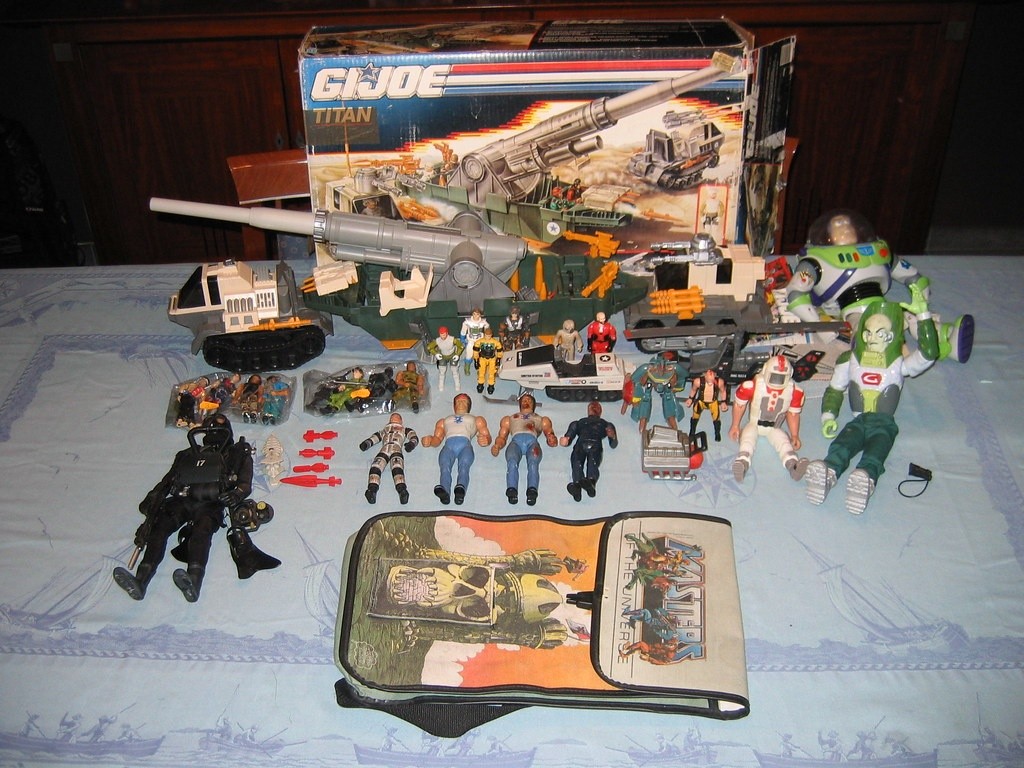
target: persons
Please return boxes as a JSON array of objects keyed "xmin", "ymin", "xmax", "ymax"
[
  {"xmin": 176, "ymin": 373, "xmax": 289, "ymax": 427},
  {"xmin": 805, "ymin": 284, "xmax": 938, "ymax": 514},
  {"xmin": 621, "ymin": 353, "xmax": 688, "ymax": 432},
  {"xmin": 360, "ymin": 413, "xmax": 419, "ymax": 504},
  {"xmin": 786, "ymin": 209, "xmax": 974, "ymax": 364},
  {"xmin": 307, "ymin": 363, "xmax": 423, "ymax": 414},
  {"xmin": 559, "ymin": 401, "xmax": 618, "ymax": 502},
  {"xmin": 492, "ymin": 395, "xmax": 557, "ymax": 506},
  {"xmin": 685, "ymin": 369, "xmax": 729, "ymax": 442},
  {"xmin": 114, "ymin": 414, "xmax": 252, "ymax": 602},
  {"xmin": 426, "ymin": 306, "xmax": 617, "ymax": 394},
  {"xmin": 729, "ymin": 355, "xmax": 809, "ymax": 483},
  {"xmin": 422, "ymin": 393, "xmax": 492, "ymax": 505}
]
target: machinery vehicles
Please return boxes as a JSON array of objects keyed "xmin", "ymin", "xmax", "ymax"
[
  {"xmin": 146, "ymin": 195, "xmax": 851, "ymax": 404},
  {"xmin": 325, "ymin": 50, "xmax": 743, "ymax": 248}
]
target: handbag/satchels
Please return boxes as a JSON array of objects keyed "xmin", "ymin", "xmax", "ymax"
[{"xmin": 335, "ymin": 511, "xmax": 748, "ymax": 737}]
